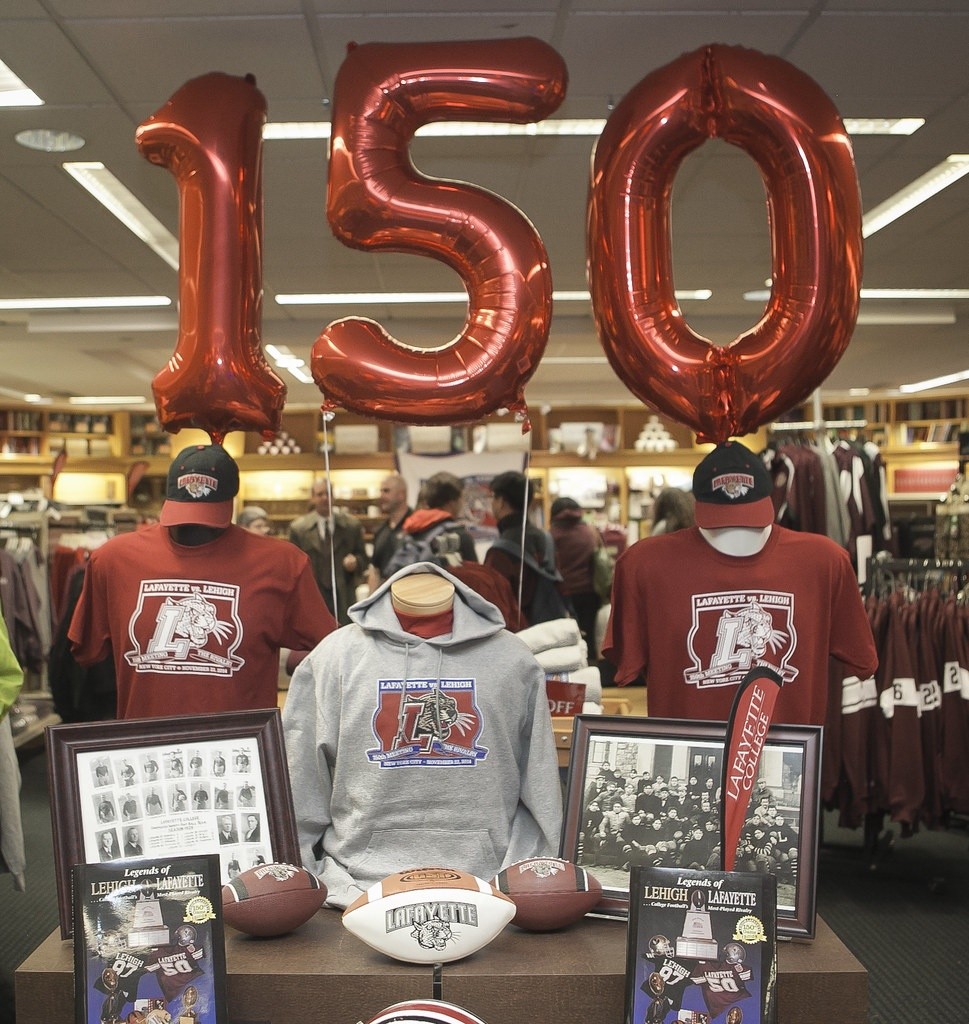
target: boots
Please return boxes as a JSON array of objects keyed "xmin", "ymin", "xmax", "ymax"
[{"xmin": 589, "ymin": 850, "xmax": 601, "ymax": 866}]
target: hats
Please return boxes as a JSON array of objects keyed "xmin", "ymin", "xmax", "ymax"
[
  {"xmin": 692, "ymin": 440, "xmax": 775, "ymax": 530},
  {"xmin": 550, "ymin": 497, "xmax": 582, "ymax": 521},
  {"xmin": 160, "ymin": 445, "xmax": 239, "ymax": 530},
  {"xmin": 756, "ymin": 778, "xmax": 766, "ymax": 783},
  {"xmin": 660, "ymin": 787, "xmax": 669, "ymax": 791},
  {"xmin": 667, "ymin": 806, "xmax": 678, "ymax": 813}
]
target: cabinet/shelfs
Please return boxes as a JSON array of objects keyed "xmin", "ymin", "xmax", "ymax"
[
  {"xmin": 0, "ymin": 402, "xmax": 765, "ymax": 528},
  {"xmin": 806, "ymin": 383, "xmax": 969, "ymax": 462}
]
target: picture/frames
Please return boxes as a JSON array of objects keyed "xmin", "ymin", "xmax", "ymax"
[
  {"xmin": 41, "ymin": 704, "xmax": 307, "ymax": 939},
  {"xmin": 562, "ymin": 709, "xmax": 843, "ymax": 945}
]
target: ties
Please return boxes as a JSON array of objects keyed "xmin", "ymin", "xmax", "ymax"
[{"xmin": 324, "ymin": 521, "xmax": 331, "ymax": 544}]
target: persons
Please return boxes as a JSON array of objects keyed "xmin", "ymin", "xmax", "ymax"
[
  {"xmin": 95, "ymin": 746, "xmax": 263, "ymax": 880},
  {"xmin": 576, "ymin": 762, "xmax": 799, "ymax": 885},
  {"xmin": 239, "ymin": 469, "xmax": 694, "ymax": 660},
  {"xmin": 618, "ymin": 441, "xmax": 877, "ymax": 727},
  {"xmin": 281, "ymin": 565, "xmax": 564, "ymax": 907},
  {"xmin": 68, "ymin": 445, "xmax": 341, "ymax": 720}
]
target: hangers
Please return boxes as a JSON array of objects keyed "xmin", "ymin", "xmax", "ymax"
[
  {"xmin": 770, "ymin": 421, "xmax": 867, "ymax": 448},
  {"xmin": 1, "ymin": 519, "xmax": 47, "ymax": 554},
  {"xmin": 855, "ymin": 554, "xmax": 969, "ymax": 610}
]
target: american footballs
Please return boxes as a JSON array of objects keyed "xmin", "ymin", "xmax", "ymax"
[
  {"xmin": 488, "ymin": 856, "xmax": 603, "ymax": 932},
  {"xmin": 222, "ymin": 862, "xmax": 329, "ymax": 937},
  {"xmin": 341, "ymin": 866, "xmax": 517, "ymax": 965}
]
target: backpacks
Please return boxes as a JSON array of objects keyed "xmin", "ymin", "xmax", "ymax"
[
  {"xmin": 383, "ymin": 521, "xmax": 465, "ymax": 578},
  {"xmin": 488, "ymin": 529, "xmax": 572, "ymax": 625}
]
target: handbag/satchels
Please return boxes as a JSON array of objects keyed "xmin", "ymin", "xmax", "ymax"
[{"xmin": 588, "ymin": 524, "xmax": 613, "ymax": 604}]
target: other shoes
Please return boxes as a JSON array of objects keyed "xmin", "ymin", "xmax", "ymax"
[{"xmin": 622, "ymin": 861, "xmax": 630, "ymax": 872}]
